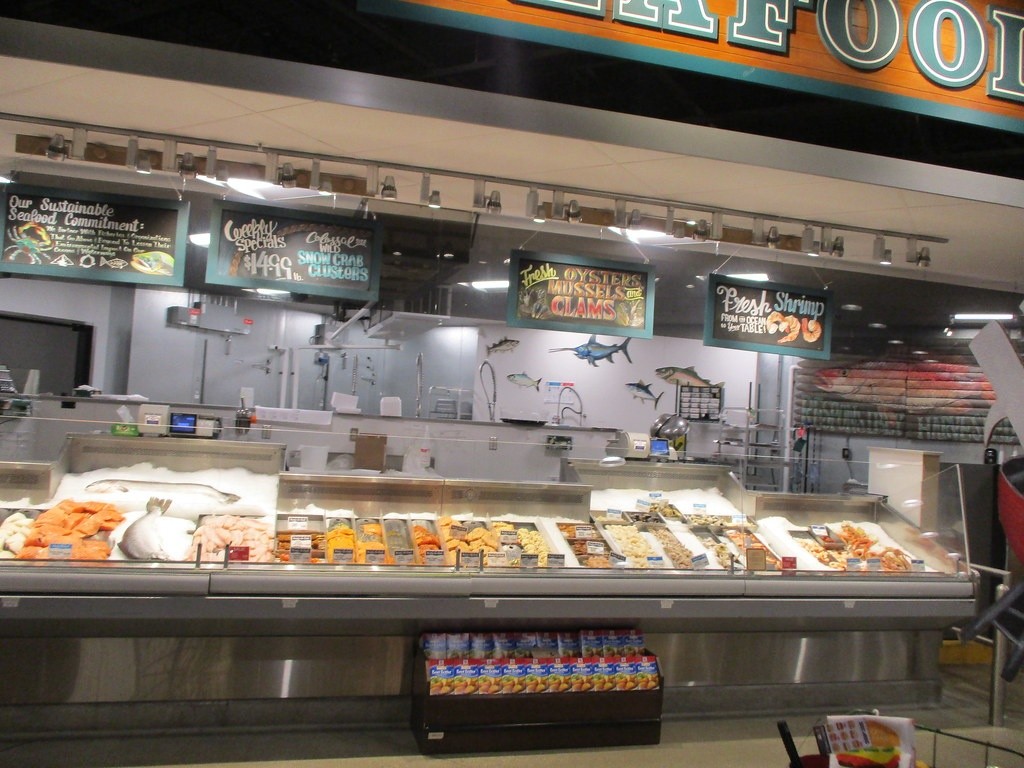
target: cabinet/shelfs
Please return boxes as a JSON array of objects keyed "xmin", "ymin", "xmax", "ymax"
[{"xmin": 711, "ymin": 381, "xmax": 785, "ymax": 492}]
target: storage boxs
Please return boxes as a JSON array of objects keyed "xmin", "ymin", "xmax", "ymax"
[{"xmin": 410, "ymin": 632, "xmax": 665, "ymax": 755}]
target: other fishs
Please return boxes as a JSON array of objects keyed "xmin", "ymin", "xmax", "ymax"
[
  {"xmin": 507, "ymin": 373, "xmax": 542, "ymax": 391},
  {"xmin": 130, "ymin": 251, "xmax": 174, "ymax": 275},
  {"xmin": 85, "ymin": 479, "xmax": 241, "ymax": 506},
  {"xmin": 487, "ymin": 336, "xmax": 519, "ymax": 357},
  {"xmin": 625, "ymin": 379, "xmax": 664, "ymax": 409},
  {"xmin": 656, "ymin": 366, "xmax": 724, "ymax": 390},
  {"xmin": 117, "ymin": 497, "xmax": 172, "ymax": 560}
]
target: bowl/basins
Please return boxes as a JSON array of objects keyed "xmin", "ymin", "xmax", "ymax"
[{"xmin": 650, "ymin": 413, "xmax": 689, "ymax": 440}]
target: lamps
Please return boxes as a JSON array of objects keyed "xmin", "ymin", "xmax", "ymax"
[
  {"xmin": 44, "ymin": 134, "xmax": 152, "ymax": 175},
  {"xmin": 376, "ymin": 175, "xmax": 397, "ymax": 198},
  {"xmin": 906, "ymin": 239, "xmax": 931, "ymax": 268},
  {"xmin": 533, "ymin": 204, "xmax": 546, "ymax": 223},
  {"xmin": 693, "ymin": 219, "xmax": 708, "ymax": 242},
  {"xmin": 204, "ymin": 165, "xmax": 228, "ymax": 182},
  {"xmin": 629, "ymin": 207, "xmax": 640, "ymax": 230},
  {"xmin": 561, "ymin": 199, "xmax": 582, "ymax": 225},
  {"xmin": 484, "ymin": 190, "xmax": 501, "ymax": 216},
  {"xmin": 767, "ymin": 225, "xmax": 781, "ymax": 249},
  {"xmin": 872, "ymin": 234, "xmax": 891, "ymax": 266},
  {"xmin": 800, "ymin": 223, "xmax": 815, "ymax": 253},
  {"xmin": 830, "ymin": 236, "xmax": 845, "ymax": 257},
  {"xmin": 275, "ymin": 163, "xmax": 297, "ymax": 189},
  {"xmin": 424, "ymin": 190, "xmax": 442, "ymax": 208},
  {"xmin": 176, "ymin": 151, "xmax": 197, "ymax": 181}
]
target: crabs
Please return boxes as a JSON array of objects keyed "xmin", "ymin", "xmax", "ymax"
[{"xmin": 4, "ymin": 222, "xmax": 53, "ymax": 265}]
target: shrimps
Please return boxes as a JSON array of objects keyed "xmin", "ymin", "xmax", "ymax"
[{"xmin": 767, "ymin": 312, "xmax": 822, "ymax": 343}]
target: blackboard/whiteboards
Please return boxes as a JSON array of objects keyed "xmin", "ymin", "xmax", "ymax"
[
  {"xmin": 0, "ymin": 183, "xmax": 191, "ymax": 287},
  {"xmin": 706, "ymin": 274, "xmax": 832, "ymax": 360},
  {"xmin": 203, "ymin": 198, "xmax": 381, "ymax": 301},
  {"xmin": 506, "ymin": 249, "xmax": 654, "ymax": 339}
]
